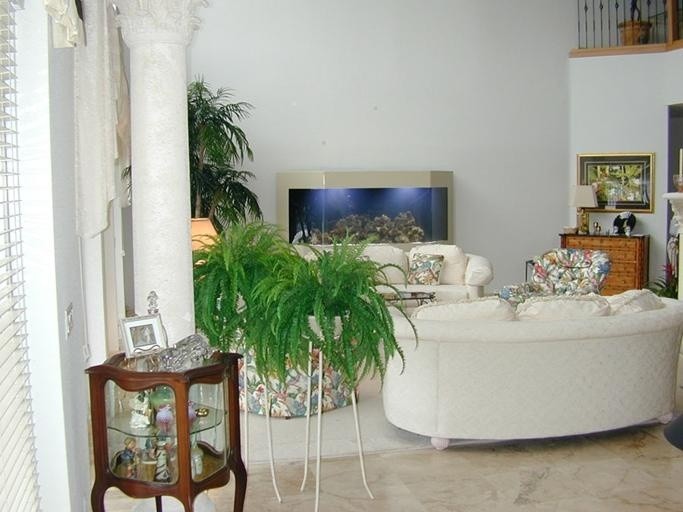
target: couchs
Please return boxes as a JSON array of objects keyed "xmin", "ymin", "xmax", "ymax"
[
  {"xmin": 499, "ymin": 248, "xmax": 611, "ymax": 300},
  {"xmin": 366, "ymin": 245, "xmax": 493, "ymax": 300},
  {"xmin": 383, "ymin": 289, "xmax": 683, "ymax": 450}
]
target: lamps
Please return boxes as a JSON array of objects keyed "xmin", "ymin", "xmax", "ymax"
[{"xmin": 567, "ymin": 185, "xmax": 599, "ymax": 236}]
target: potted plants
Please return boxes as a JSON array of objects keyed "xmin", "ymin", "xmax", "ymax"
[
  {"xmin": 185, "ymin": 73, "xmax": 263, "ymax": 249},
  {"xmin": 618, "ymin": 0, "xmax": 654, "ymax": 47},
  {"xmin": 246, "ymin": 235, "xmax": 417, "ymax": 510}
]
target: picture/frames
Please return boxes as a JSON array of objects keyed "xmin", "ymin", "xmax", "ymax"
[
  {"xmin": 575, "ymin": 151, "xmax": 657, "ymax": 215},
  {"xmin": 115, "ymin": 313, "xmax": 169, "ymax": 358}
]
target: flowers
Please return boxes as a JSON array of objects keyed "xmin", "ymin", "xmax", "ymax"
[{"xmin": 651, "ymin": 261, "xmax": 680, "ymax": 299}]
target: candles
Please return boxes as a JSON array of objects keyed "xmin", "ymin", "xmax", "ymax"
[{"xmin": 679, "ymin": 148, "xmax": 682, "ymax": 177}]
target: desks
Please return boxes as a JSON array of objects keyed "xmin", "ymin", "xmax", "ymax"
[{"xmin": 82, "ymin": 348, "xmax": 248, "ymax": 511}]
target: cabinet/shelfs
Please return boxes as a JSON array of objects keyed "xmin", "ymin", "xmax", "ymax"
[{"xmin": 560, "ymin": 231, "xmax": 651, "ymax": 295}]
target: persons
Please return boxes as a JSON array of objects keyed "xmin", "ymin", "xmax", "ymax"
[{"xmin": 121, "ymin": 438, "xmax": 137, "ymax": 478}]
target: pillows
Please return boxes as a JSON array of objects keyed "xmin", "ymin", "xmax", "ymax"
[{"xmin": 407, "ymin": 252, "xmax": 444, "ymax": 286}]
